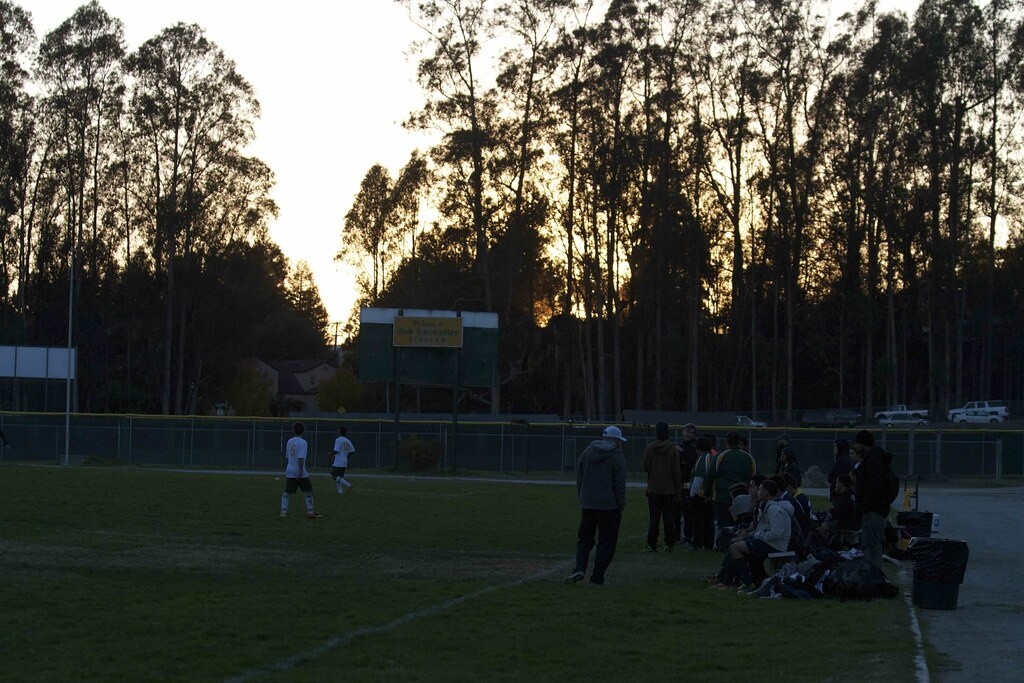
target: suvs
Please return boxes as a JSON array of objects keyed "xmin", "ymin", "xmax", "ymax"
[{"xmin": 800, "ymin": 409, "xmax": 855, "ymax": 429}]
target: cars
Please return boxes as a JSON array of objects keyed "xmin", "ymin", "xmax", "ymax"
[
  {"xmin": 735, "ymin": 415, "xmax": 765, "ymax": 427},
  {"xmin": 952, "ymin": 409, "xmax": 1004, "ymax": 426},
  {"xmin": 881, "ymin": 412, "xmax": 929, "ymax": 429}
]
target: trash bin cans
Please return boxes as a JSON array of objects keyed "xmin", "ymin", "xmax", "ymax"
[
  {"xmin": 911, "ymin": 537, "xmax": 967, "ymax": 611},
  {"xmin": 898, "ymin": 510, "xmax": 932, "ymax": 559}
]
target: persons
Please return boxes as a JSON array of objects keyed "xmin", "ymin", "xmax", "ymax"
[
  {"xmin": 278, "ymin": 422, "xmax": 322, "ymax": 519},
  {"xmin": 328, "ymin": 427, "xmax": 355, "ymax": 495},
  {"xmin": 567, "ymin": 426, "xmax": 629, "ymax": 586},
  {"xmin": 642, "ymin": 421, "xmax": 900, "ymax": 594},
  {"xmin": 0, "ymin": 428, "xmax": 11, "ymax": 450}
]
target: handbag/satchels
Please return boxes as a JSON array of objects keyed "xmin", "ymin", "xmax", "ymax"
[{"xmin": 879, "ymin": 455, "xmax": 899, "ymax": 503}]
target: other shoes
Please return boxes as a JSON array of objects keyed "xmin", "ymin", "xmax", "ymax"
[
  {"xmin": 642, "ymin": 536, "xmax": 731, "ymax": 553},
  {"xmin": 307, "ymin": 511, "xmax": 322, "ymax": 519},
  {"xmin": 590, "ymin": 581, "xmax": 610, "ymax": 588},
  {"xmin": 347, "ymin": 484, "xmax": 352, "ymax": 495},
  {"xmin": 280, "ymin": 512, "xmax": 288, "ymax": 517},
  {"xmin": 565, "ymin": 570, "xmax": 584, "ymax": 583}
]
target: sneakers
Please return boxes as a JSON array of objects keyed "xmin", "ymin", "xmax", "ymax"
[
  {"xmin": 735, "ymin": 582, "xmax": 755, "ymax": 594},
  {"xmin": 714, "ymin": 582, "xmax": 733, "ymax": 590},
  {"xmin": 704, "ymin": 574, "xmax": 720, "ymax": 584}
]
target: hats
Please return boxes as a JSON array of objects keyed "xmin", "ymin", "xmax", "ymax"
[
  {"xmin": 852, "ymin": 429, "xmax": 875, "ymax": 447},
  {"xmin": 776, "ymin": 433, "xmax": 790, "ymax": 442},
  {"xmin": 656, "ymin": 421, "xmax": 669, "ymax": 441},
  {"xmin": 603, "ymin": 426, "xmax": 627, "ymax": 441}
]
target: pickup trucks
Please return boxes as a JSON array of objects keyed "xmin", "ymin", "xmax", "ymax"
[
  {"xmin": 946, "ymin": 400, "xmax": 1010, "ymax": 422},
  {"xmin": 874, "ymin": 404, "xmax": 929, "ymax": 421}
]
